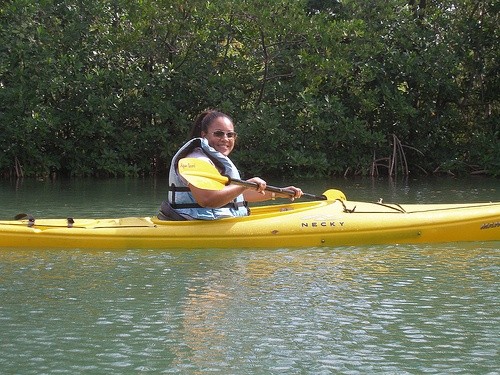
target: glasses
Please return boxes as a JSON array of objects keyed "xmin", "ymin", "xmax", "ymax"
[{"xmin": 206, "ymin": 130, "xmax": 238, "ymax": 139}]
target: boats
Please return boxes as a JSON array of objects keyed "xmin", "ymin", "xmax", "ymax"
[{"xmin": 0, "ymin": 187, "xmax": 499, "ymax": 247}]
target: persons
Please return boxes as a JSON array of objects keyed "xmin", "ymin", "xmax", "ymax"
[{"xmin": 168, "ymin": 110, "xmax": 304, "ymax": 221}]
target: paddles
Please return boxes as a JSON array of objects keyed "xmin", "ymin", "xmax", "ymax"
[{"xmin": 178, "ymin": 158, "xmax": 347, "ymax": 205}]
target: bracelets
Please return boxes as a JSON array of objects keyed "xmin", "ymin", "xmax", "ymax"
[{"xmin": 271, "ymin": 192, "xmax": 276, "ymax": 201}]
